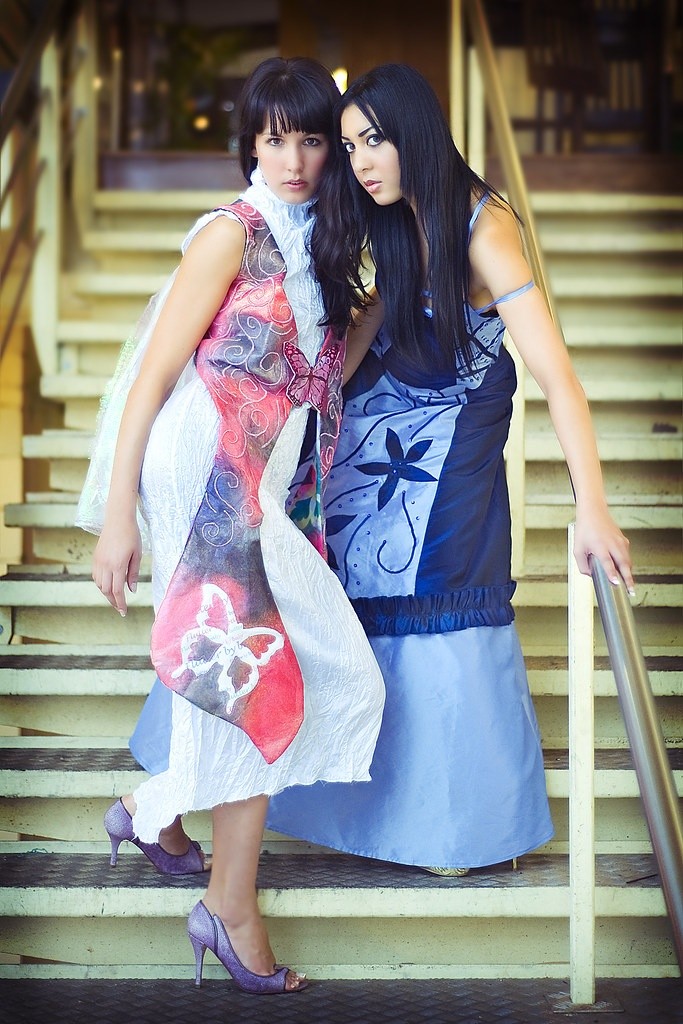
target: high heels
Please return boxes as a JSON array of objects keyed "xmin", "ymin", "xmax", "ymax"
[
  {"xmin": 422, "ymin": 856, "xmax": 519, "ymax": 877},
  {"xmin": 104, "ymin": 797, "xmax": 209, "ymax": 880},
  {"xmin": 186, "ymin": 901, "xmax": 308, "ymax": 995}
]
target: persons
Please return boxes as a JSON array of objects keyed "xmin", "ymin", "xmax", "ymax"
[
  {"xmin": 128, "ymin": 64, "xmax": 637, "ymax": 874},
  {"xmin": 76, "ymin": 54, "xmax": 387, "ymax": 994}
]
